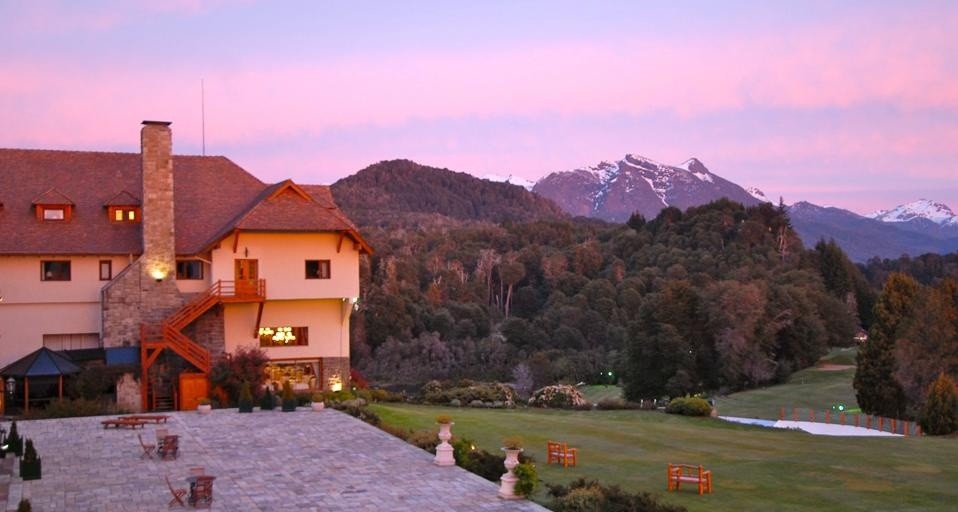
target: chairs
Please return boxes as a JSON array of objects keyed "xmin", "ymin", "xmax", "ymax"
[
  {"xmin": 163, "ymin": 465, "xmax": 218, "ymax": 509},
  {"xmin": 136, "ymin": 427, "xmax": 181, "ymax": 461}
]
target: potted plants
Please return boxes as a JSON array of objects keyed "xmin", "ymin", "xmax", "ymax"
[
  {"xmin": 197, "ymin": 380, "xmax": 325, "ymax": 414},
  {"xmin": 4, "ymin": 417, "xmax": 41, "ymax": 481},
  {"xmin": 434, "ymin": 415, "xmax": 455, "ymax": 440},
  {"xmin": 499, "ymin": 435, "xmax": 525, "ymax": 470}
]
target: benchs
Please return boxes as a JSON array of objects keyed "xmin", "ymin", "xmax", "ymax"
[
  {"xmin": 548, "ymin": 440, "xmax": 577, "ymax": 469},
  {"xmin": 667, "ymin": 463, "xmax": 712, "ymax": 496},
  {"xmin": 101, "ymin": 415, "xmax": 170, "ymax": 430}
]
